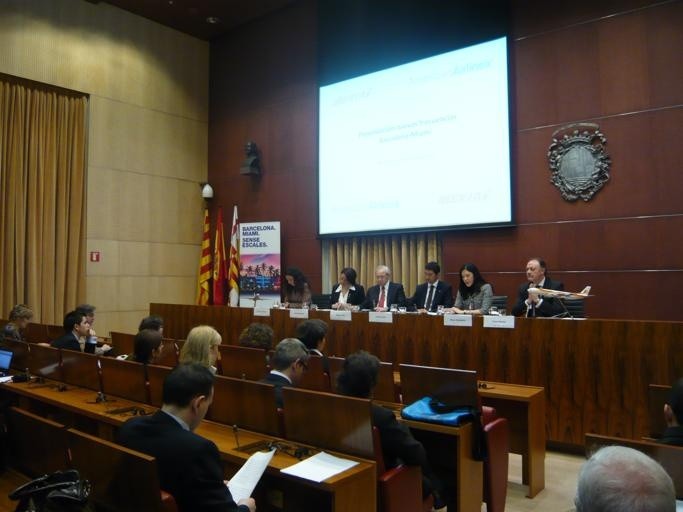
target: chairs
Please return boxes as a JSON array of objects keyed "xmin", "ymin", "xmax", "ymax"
[{"xmin": 310, "ymin": 294, "xmax": 586, "ymax": 318}]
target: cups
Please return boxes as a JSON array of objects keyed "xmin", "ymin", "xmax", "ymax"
[
  {"xmin": 437, "ymin": 304, "xmax": 444, "ymax": 312},
  {"xmin": 488, "ymin": 306, "xmax": 505, "ymax": 317}
]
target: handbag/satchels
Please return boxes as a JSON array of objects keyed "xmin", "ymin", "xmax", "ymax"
[
  {"xmin": 8, "ymin": 467, "xmax": 93, "ymax": 512},
  {"xmin": 399, "ymin": 394, "xmax": 481, "ymax": 428}
]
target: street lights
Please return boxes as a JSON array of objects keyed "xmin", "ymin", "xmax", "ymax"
[{"xmin": 199, "ymin": 177, "xmax": 218, "ymax": 299}]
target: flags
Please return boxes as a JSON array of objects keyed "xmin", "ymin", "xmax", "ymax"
[
  {"xmin": 209, "ymin": 207, "xmax": 228, "ymax": 307},
  {"xmin": 227, "ymin": 205, "xmax": 240, "ymax": 308},
  {"xmin": 194, "ymin": 208, "xmax": 213, "ymax": 306}
]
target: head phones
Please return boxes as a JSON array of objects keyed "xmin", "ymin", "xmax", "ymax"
[
  {"xmin": 478, "ymin": 377, "xmax": 495, "ymax": 392},
  {"xmin": 130, "ymin": 403, "xmax": 157, "ymax": 416},
  {"xmin": 270, "ymin": 436, "xmax": 311, "ymax": 458}
]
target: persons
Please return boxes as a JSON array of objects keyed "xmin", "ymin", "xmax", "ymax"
[
  {"xmin": 654, "ymin": 378, "xmax": 682, "ymax": 447},
  {"xmin": 131, "ymin": 314, "xmax": 221, "ymax": 367},
  {"xmin": 49, "ymin": 304, "xmax": 111, "ymax": 354},
  {"xmin": 443, "ymin": 262, "xmax": 494, "ymax": 315},
  {"xmin": 117, "ymin": 361, "xmax": 259, "ymax": 511},
  {"xmin": 333, "ymin": 350, "xmax": 446, "ymax": 510},
  {"xmin": 239, "ymin": 318, "xmax": 328, "ymax": 389},
  {"xmin": 3, "ymin": 304, "xmax": 33, "ymax": 344},
  {"xmin": 241, "ymin": 141, "xmax": 258, "ymax": 167},
  {"xmin": 405, "ymin": 262, "xmax": 452, "ymax": 313},
  {"xmin": 349, "ymin": 265, "xmax": 405, "ymax": 312},
  {"xmin": 325, "ymin": 268, "xmax": 366, "ymax": 310},
  {"xmin": 574, "ymin": 443, "xmax": 675, "ymax": 511},
  {"xmin": 279, "ymin": 265, "xmax": 312, "ymax": 308},
  {"xmin": 510, "ymin": 257, "xmax": 564, "ymax": 317}
]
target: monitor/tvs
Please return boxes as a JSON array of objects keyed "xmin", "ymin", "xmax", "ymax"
[{"xmin": 311, "ymin": 294, "xmax": 333, "ymax": 309}]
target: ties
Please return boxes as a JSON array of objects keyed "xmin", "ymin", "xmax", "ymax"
[
  {"xmin": 425, "ymin": 285, "xmax": 435, "ymax": 311},
  {"xmin": 378, "ymin": 287, "xmax": 385, "ymax": 308},
  {"xmin": 527, "ymin": 284, "xmax": 541, "ymax": 319}
]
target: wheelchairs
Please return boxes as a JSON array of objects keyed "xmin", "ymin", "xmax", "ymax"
[{"xmin": 7, "ymin": 446, "xmax": 98, "ymax": 512}]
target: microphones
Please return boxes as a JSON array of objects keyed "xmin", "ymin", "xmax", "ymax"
[
  {"xmin": 231, "ymin": 423, "xmax": 246, "ymax": 451},
  {"xmin": 370, "ymin": 295, "xmax": 377, "ymax": 311}
]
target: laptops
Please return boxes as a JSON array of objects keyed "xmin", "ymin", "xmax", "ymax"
[{"xmin": 1, "ymin": 349, "xmax": 14, "ymax": 376}]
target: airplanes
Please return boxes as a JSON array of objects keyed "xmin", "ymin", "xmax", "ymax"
[{"xmin": 240, "ymin": 292, "xmax": 272, "ymax": 303}]
